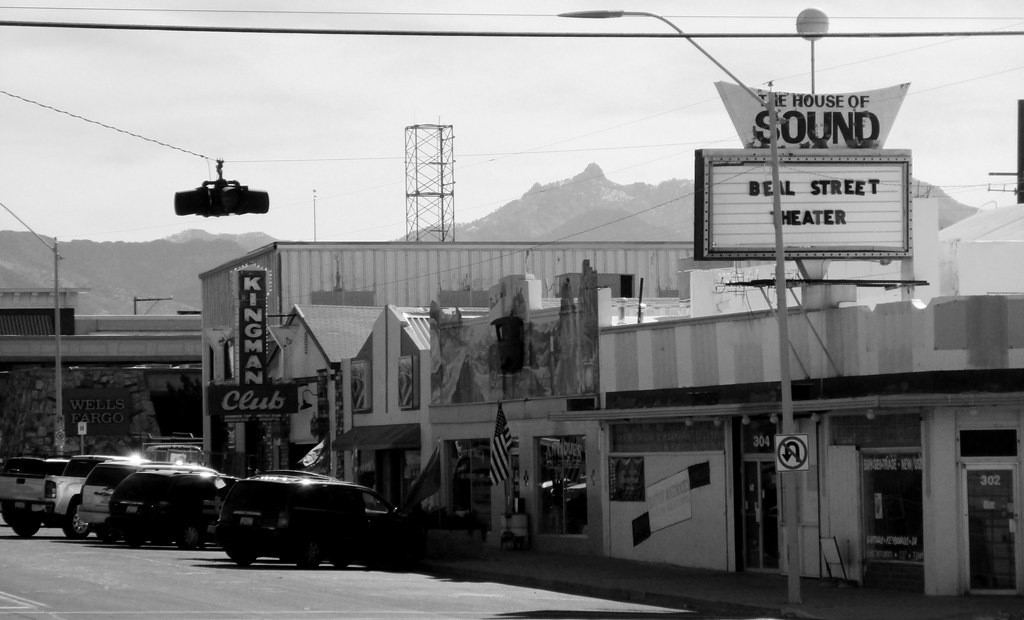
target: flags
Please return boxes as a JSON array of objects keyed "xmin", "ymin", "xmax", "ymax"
[
  {"xmin": 484, "ymin": 400, "xmax": 514, "ymax": 486},
  {"xmin": 402, "ymin": 435, "xmax": 442, "ymax": 515},
  {"xmin": 296, "ymin": 433, "xmax": 330, "ymax": 477}
]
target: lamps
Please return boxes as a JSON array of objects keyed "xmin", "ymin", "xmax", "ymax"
[
  {"xmin": 811, "ymin": 412, "xmax": 820, "ymax": 422},
  {"xmin": 742, "ymin": 414, "xmax": 751, "ymax": 425},
  {"xmin": 684, "ymin": 417, "xmax": 693, "ymax": 426},
  {"xmin": 714, "ymin": 416, "xmax": 722, "ymax": 426},
  {"xmin": 866, "ymin": 409, "xmax": 876, "ymax": 420},
  {"xmin": 300, "ymin": 389, "xmax": 323, "ymax": 411},
  {"xmin": 969, "ymin": 406, "xmax": 979, "ymax": 417},
  {"xmin": 769, "ymin": 412, "xmax": 779, "ymax": 424}
]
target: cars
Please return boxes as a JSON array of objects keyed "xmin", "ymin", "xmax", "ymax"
[
  {"xmin": 51, "ymin": 454, "xmax": 139, "ymax": 538},
  {"xmin": 81, "ymin": 460, "xmax": 218, "ymax": 544},
  {"xmin": 212, "ymin": 469, "xmax": 417, "ymax": 571},
  {"xmin": 108, "ymin": 465, "xmax": 238, "ymax": 550},
  {"xmin": 0, "ymin": 455, "xmax": 67, "ymax": 537}
]
